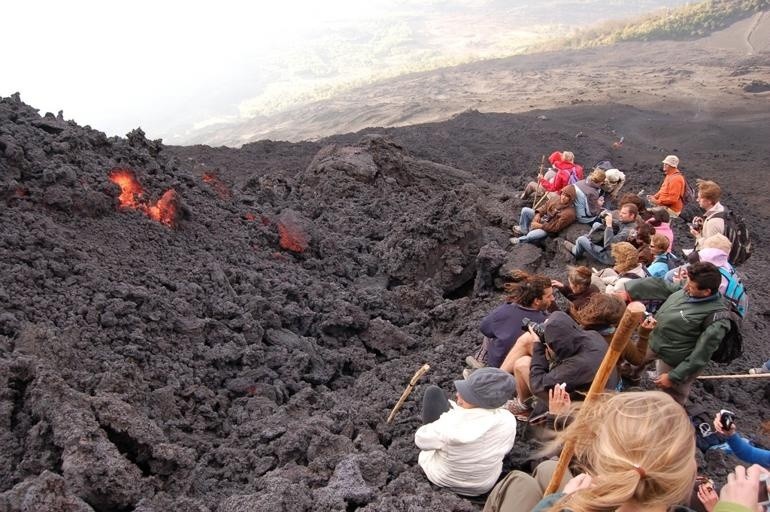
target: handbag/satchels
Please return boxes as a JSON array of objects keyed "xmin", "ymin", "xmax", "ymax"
[{"xmin": 590, "ymin": 221, "xmax": 620, "ymax": 248}]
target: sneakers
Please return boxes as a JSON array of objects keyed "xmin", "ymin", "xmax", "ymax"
[
  {"xmin": 510, "ymin": 238, "xmax": 519, "ymax": 244},
  {"xmin": 563, "ymin": 240, "xmax": 575, "ymax": 252},
  {"xmin": 516, "ymin": 191, "xmax": 529, "ymax": 200},
  {"xmin": 749, "ymin": 367, "xmax": 763, "ymax": 374},
  {"xmin": 513, "ymin": 226, "xmax": 524, "ymax": 234}
]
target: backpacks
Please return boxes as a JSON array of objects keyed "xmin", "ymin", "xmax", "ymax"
[
  {"xmin": 564, "ymin": 167, "xmax": 579, "ymax": 185},
  {"xmin": 674, "ymin": 173, "xmax": 695, "ymax": 205},
  {"xmin": 711, "ymin": 206, "xmax": 752, "ymax": 265},
  {"xmin": 656, "ymin": 252, "xmax": 685, "ymax": 270},
  {"xmin": 703, "ymin": 301, "xmax": 742, "ymax": 363},
  {"xmin": 718, "ymin": 263, "xmax": 749, "ymax": 320}
]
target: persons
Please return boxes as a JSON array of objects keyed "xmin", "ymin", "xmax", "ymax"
[{"xmin": 415, "ymin": 150, "xmax": 770, "ymax": 512}]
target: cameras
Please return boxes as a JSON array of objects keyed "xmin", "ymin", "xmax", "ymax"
[
  {"xmin": 687, "ymin": 221, "xmax": 699, "ymax": 231},
  {"xmin": 722, "ymin": 412, "xmax": 736, "ymax": 431},
  {"xmin": 601, "ymin": 210, "xmax": 608, "ymax": 218},
  {"xmin": 520, "ymin": 317, "xmax": 544, "ymax": 342}
]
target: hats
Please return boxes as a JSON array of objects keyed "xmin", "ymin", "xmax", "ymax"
[
  {"xmin": 455, "ymin": 367, "xmax": 516, "ymax": 410},
  {"xmin": 662, "ymin": 155, "xmax": 679, "ymax": 168}
]
